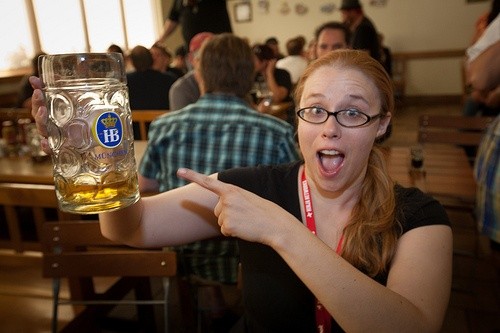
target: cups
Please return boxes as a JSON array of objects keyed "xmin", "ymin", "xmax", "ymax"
[{"xmin": 36, "ymin": 52, "xmax": 140, "ymax": 214}]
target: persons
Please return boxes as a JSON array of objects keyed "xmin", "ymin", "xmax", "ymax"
[
  {"xmin": 29, "ymin": 49, "xmax": 451, "ymax": 333},
  {"xmin": 18, "ymin": 0, "xmax": 500, "ymax": 279}
]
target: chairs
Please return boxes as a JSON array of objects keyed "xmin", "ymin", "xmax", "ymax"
[
  {"xmin": 0, "ymin": 98, "xmax": 293, "ymax": 333},
  {"xmin": 411, "ymin": 115, "xmax": 493, "ymax": 278}
]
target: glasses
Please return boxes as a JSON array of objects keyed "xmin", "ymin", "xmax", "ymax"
[{"xmin": 297, "ymin": 106, "xmax": 381, "ymax": 127}]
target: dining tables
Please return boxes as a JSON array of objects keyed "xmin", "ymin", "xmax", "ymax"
[{"xmin": 0, "ymin": 135, "xmax": 150, "ymax": 186}]
